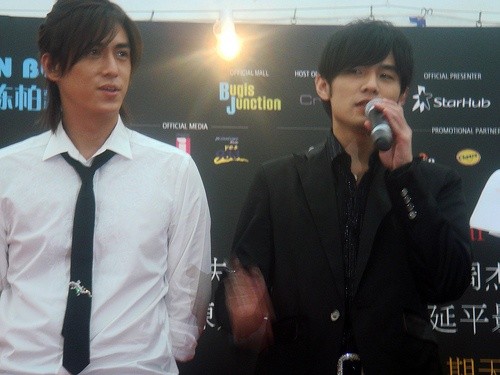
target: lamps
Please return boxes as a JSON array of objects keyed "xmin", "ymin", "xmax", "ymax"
[{"xmin": 212, "ymin": 11, "xmax": 242, "ymax": 62}]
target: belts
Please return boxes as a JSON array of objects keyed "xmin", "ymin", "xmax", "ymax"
[{"xmin": 336, "ymin": 354, "xmax": 363, "ymax": 375}]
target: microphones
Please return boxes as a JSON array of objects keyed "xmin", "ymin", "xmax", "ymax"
[{"xmin": 364, "ymin": 97, "xmax": 394, "ymax": 152}]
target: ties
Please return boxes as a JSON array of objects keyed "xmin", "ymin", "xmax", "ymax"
[{"xmin": 61, "ymin": 149, "xmax": 117, "ymax": 375}]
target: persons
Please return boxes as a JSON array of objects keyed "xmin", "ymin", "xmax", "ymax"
[
  {"xmin": 213, "ymin": 19, "xmax": 473, "ymax": 375},
  {"xmin": 0, "ymin": 0, "xmax": 211, "ymax": 375}
]
